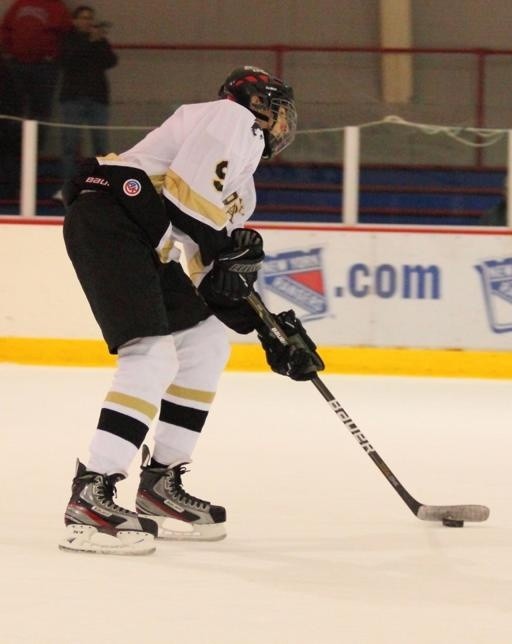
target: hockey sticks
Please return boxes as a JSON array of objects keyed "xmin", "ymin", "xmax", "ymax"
[{"xmin": 245, "ymin": 292, "xmax": 490, "ymax": 522}]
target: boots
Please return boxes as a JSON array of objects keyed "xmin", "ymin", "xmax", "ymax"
[
  {"xmin": 65, "ymin": 457, "xmax": 158, "ymax": 539},
  {"xmin": 135, "ymin": 444, "xmax": 226, "ymax": 525}
]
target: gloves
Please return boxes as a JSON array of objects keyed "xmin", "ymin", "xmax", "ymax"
[
  {"xmin": 214, "ymin": 228, "xmax": 264, "ymax": 300},
  {"xmin": 257, "ymin": 310, "xmax": 325, "ymax": 381}
]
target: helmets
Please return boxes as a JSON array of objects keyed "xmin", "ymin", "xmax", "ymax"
[{"xmin": 218, "ymin": 65, "xmax": 294, "ymax": 99}]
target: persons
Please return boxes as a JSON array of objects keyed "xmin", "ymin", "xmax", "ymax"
[
  {"xmin": 52, "ymin": 6, "xmax": 120, "ymax": 186},
  {"xmin": 63, "ymin": 65, "xmax": 327, "ymax": 537}
]
